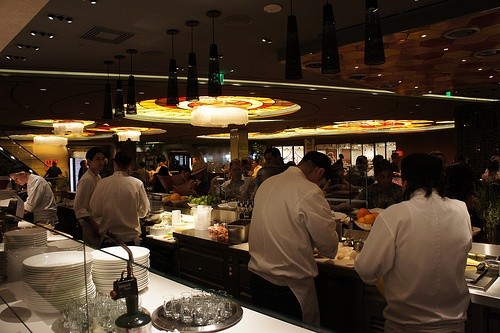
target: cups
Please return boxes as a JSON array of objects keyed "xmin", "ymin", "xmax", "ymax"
[
  {"xmin": 59, "ymin": 289, "xmax": 142, "ymax": 333},
  {"xmin": 163, "ymin": 287, "xmax": 234, "ymax": 326},
  {"xmin": 172, "ymin": 210, "xmax": 181, "ymax": 226},
  {"xmin": 191, "ymin": 206, "xmax": 212, "ymax": 230}
]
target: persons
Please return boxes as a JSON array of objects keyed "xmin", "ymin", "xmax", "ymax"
[
  {"xmin": 73, "ymin": 148, "xmax": 106, "ymax": 249},
  {"xmin": 44, "ymin": 161, "xmax": 62, "ymax": 191},
  {"xmin": 8, "ymin": 164, "xmax": 59, "ymax": 231},
  {"xmin": 89, "ymin": 153, "xmax": 150, "ymax": 249},
  {"xmin": 322, "ymin": 151, "xmax": 500, "ymax": 209},
  {"xmin": 100, "ymin": 147, "xmax": 296, "ymax": 202},
  {"xmin": 247, "ymin": 150, "xmax": 339, "ymax": 329},
  {"xmin": 78, "ymin": 160, "xmax": 88, "ymax": 182},
  {"xmin": 354, "ymin": 152, "xmax": 473, "ymax": 333}
]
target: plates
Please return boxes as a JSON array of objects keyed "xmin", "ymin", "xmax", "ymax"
[
  {"xmin": 47, "ymin": 230, "xmax": 84, "ymax": 250},
  {"xmin": 170, "ymin": 196, "xmax": 217, "ymax": 208},
  {"xmin": 0, "ymin": 227, "xmax": 48, "ymax": 281},
  {"xmin": 90, "ymin": 246, "xmax": 152, "ymax": 294},
  {"xmin": 21, "ymin": 250, "xmax": 97, "ymax": 313}
]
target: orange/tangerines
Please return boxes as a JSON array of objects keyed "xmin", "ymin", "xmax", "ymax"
[{"xmin": 358, "ymin": 213, "xmax": 379, "ymax": 226}]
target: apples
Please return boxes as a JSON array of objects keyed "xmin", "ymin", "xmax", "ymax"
[
  {"xmin": 189, "ymin": 196, "xmax": 218, "ymax": 205},
  {"xmin": 357, "ymin": 208, "xmax": 370, "ymax": 219},
  {"xmin": 162, "ymin": 193, "xmax": 188, "ymax": 203}
]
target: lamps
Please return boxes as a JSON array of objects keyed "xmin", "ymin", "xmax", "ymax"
[
  {"xmin": 206, "ymin": 10, "xmax": 222, "ymax": 98},
  {"xmin": 6, "ymin": 55, "xmax": 10, "ymax": 60},
  {"xmin": 32, "ymin": 46, "xmax": 40, "ymax": 51},
  {"xmin": 262, "ymin": 39, "xmax": 266, "ymax": 43},
  {"xmin": 264, "ymin": 4, "xmax": 283, "ymax": 13},
  {"xmin": 65, "ymin": 16, "xmax": 73, "ymax": 24},
  {"xmin": 17, "ymin": 44, "xmax": 24, "ymax": 49},
  {"xmin": 166, "ymin": 28, "xmax": 180, "ymax": 106},
  {"xmin": 102, "ymin": 61, "xmax": 114, "ymax": 121},
  {"xmin": 21, "ymin": 57, "xmax": 27, "ymax": 60},
  {"xmin": 285, "ymin": 0, "xmax": 303, "ymax": 80},
  {"xmin": 48, "ymin": 14, "xmax": 56, "ymax": 21},
  {"xmin": 363, "ymin": 0, "xmax": 386, "ymax": 65},
  {"xmin": 321, "ymin": 0, "xmax": 340, "ymax": 74},
  {"xmin": 47, "ymin": 34, "xmax": 54, "ymax": 40},
  {"xmin": 268, "ymin": 40, "xmax": 273, "ymax": 44},
  {"xmin": 33, "ymin": 136, "xmax": 68, "ymax": 146},
  {"xmin": 185, "ymin": 20, "xmax": 200, "ymax": 101},
  {"xmin": 53, "ymin": 122, "xmax": 84, "ymax": 136},
  {"xmin": 113, "ymin": 54, "xmax": 125, "ymax": 118},
  {"xmin": 190, "ymin": 104, "xmax": 248, "ymax": 128},
  {"xmin": 30, "ymin": 31, "xmax": 38, "ymax": 36},
  {"xmin": 118, "ymin": 131, "xmax": 141, "ymax": 142},
  {"xmin": 126, "ymin": 49, "xmax": 137, "ymax": 115}
]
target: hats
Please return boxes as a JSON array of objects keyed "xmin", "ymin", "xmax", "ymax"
[
  {"xmin": 400, "ymin": 153, "xmax": 444, "ymax": 182},
  {"xmin": 8, "ymin": 164, "xmax": 28, "ymax": 174},
  {"xmin": 191, "ymin": 161, "xmax": 208, "ymax": 175},
  {"xmin": 302, "ymin": 151, "xmax": 339, "ymax": 179}
]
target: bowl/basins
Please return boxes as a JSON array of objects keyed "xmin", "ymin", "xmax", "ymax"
[
  {"xmin": 472, "ymin": 226, "xmax": 481, "ymax": 236},
  {"xmin": 217, "ymin": 201, "xmax": 237, "ymax": 210},
  {"xmin": 355, "ymin": 220, "xmax": 374, "ymax": 231}
]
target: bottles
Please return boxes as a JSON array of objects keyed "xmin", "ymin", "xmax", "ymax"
[{"xmin": 236, "ymin": 198, "xmax": 254, "ymax": 219}]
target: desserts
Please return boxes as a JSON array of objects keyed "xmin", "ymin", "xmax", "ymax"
[{"xmin": 208, "ymin": 226, "xmax": 228, "ymax": 241}]
